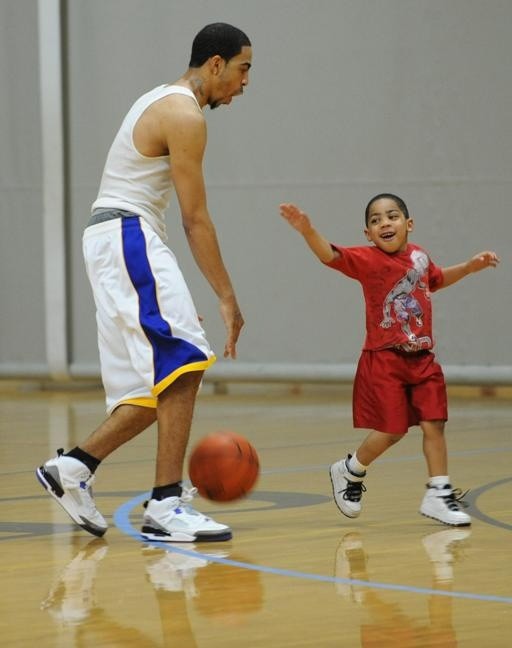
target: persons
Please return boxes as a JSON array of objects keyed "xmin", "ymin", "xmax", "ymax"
[
  {"xmin": 280, "ymin": 194, "xmax": 500, "ymax": 527},
  {"xmin": 335, "ymin": 527, "xmax": 472, "ymax": 648},
  {"xmin": 40, "ymin": 537, "xmax": 233, "ymax": 648},
  {"xmin": 36, "ymin": 22, "xmax": 253, "ymax": 543}
]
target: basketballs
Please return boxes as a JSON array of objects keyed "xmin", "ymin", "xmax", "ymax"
[{"xmin": 188, "ymin": 434, "xmax": 258, "ymax": 503}]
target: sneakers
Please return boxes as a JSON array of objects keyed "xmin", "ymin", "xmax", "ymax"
[
  {"xmin": 142, "ymin": 549, "xmax": 232, "ymax": 587},
  {"xmin": 36, "ymin": 455, "xmax": 108, "ymax": 538},
  {"xmin": 38, "ymin": 542, "xmax": 109, "ymax": 618},
  {"xmin": 330, "ymin": 455, "xmax": 367, "ymax": 519},
  {"xmin": 420, "ymin": 485, "xmax": 473, "ymax": 528},
  {"xmin": 424, "ymin": 531, "xmax": 469, "ymax": 573},
  {"xmin": 335, "ymin": 534, "xmax": 370, "ymax": 604},
  {"xmin": 144, "ymin": 498, "xmax": 233, "ymax": 543}
]
